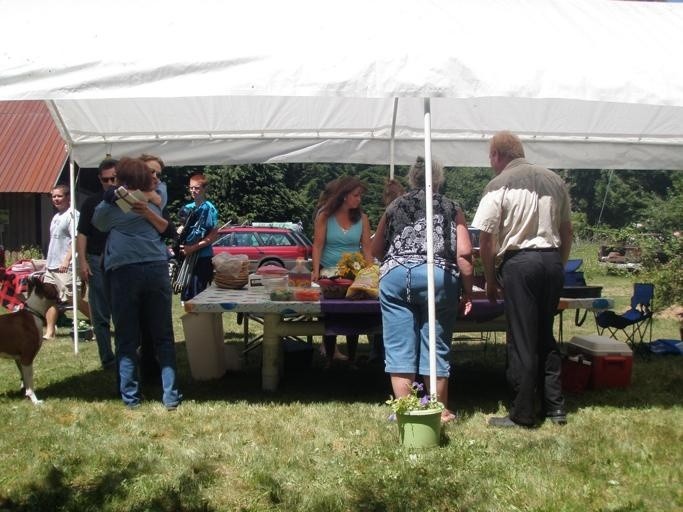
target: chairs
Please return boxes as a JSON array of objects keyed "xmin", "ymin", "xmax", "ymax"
[{"xmin": 594, "ymin": 283, "xmax": 654, "ymax": 362}]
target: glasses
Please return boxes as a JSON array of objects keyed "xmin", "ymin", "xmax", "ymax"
[
  {"xmin": 151, "ymin": 168, "xmax": 161, "ymax": 179},
  {"xmin": 100, "ymin": 176, "xmax": 116, "ymax": 184}
]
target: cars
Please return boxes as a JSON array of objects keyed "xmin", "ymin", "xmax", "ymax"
[{"xmin": 465, "ymin": 225, "xmax": 503, "ymax": 279}]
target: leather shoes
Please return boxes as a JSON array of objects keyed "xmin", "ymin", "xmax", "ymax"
[
  {"xmin": 545, "ymin": 410, "xmax": 567, "ymax": 422},
  {"xmin": 488, "ymin": 415, "xmax": 538, "ymax": 428}
]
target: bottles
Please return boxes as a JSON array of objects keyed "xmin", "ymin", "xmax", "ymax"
[{"xmin": 288, "ymin": 256, "xmax": 311, "ymax": 289}]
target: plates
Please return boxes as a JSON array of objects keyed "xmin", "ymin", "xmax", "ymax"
[{"xmin": 213, "ymin": 259, "xmax": 249, "ymax": 289}]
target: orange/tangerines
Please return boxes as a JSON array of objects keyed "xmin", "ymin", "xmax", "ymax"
[{"xmin": 270, "ymin": 288, "xmax": 292, "ymax": 301}]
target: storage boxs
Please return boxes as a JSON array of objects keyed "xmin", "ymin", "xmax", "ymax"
[{"xmin": 566, "ymin": 335, "xmax": 633, "ymax": 390}]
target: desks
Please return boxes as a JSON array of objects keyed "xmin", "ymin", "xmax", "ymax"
[{"xmin": 184, "ymin": 285, "xmax": 615, "ymax": 392}]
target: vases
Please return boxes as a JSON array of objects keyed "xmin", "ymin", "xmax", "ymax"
[
  {"xmin": 70, "ymin": 326, "xmax": 94, "ymax": 340},
  {"xmin": 396, "ymin": 402, "xmax": 444, "ymax": 454}
]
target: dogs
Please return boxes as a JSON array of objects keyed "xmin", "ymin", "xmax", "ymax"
[{"xmin": 0, "ymin": 275, "xmax": 69, "ymax": 405}]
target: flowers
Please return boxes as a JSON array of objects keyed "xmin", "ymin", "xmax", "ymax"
[
  {"xmin": 385, "ymin": 382, "xmax": 439, "ymax": 422},
  {"xmin": 77, "ymin": 319, "xmax": 89, "ymax": 330},
  {"xmin": 336, "ymin": 252, "xmax": 369, "ymax": 279}
]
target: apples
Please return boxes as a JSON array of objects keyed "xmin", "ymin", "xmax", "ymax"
[{"xmin": 292, "ymin": 289, "xmax": 321, "ymax": 301}]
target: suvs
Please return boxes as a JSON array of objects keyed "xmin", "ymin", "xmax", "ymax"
[{"xmin": 166, "ymin": 221, "xmax": 315, "ymax": 283}]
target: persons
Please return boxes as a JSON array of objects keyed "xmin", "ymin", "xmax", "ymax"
[
  {"xmin": 471, "ymin": 132, "xmax": 573, "ymax": 430},
  {"xmin": 170, "ymin": 174, "xmax": 218, "ymax": 302},
  {"xmin": 76, "ymin": 159, "xmax": 118, "ymax": 372},
  {"xmin": 313, "ymin": 177, "xmax": 372, "ymax": 370},
  {"xmin": 39, "ymin": 184, "xmax": 91, "ymax": 340},
  {"xmin": 102, "ymin": 155, "xmax": 173, "ymax": 400},
  {"xmin": 383, "ymin": 179, "xmax": 405, "ymax": 206},
  {"xmin": 371, "ymin": 155, "xmax": 474, "ymax": 426},
  {"xmin": 91, "ymin": 156, "xmax": 184, "ymax": 412}
]
